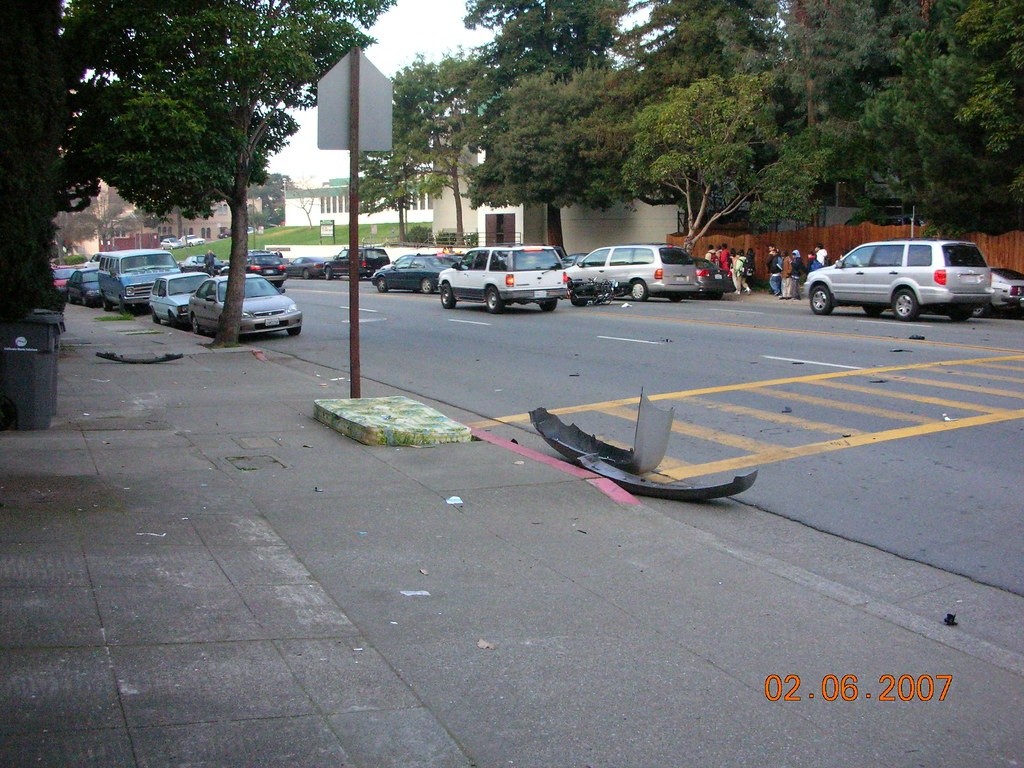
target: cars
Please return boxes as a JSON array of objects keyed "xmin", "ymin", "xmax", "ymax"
[
  {"xmin": 689, "ymin": 256, "xmax": 737, "ymax": 300},
  {"xmin": 178, "ymin": 234, "xmax": 206, "ymax": 247},
  {"xmin": 285, "ymin": 256, "xmax": 327, "ymax": 280},
  {"xmin": 160, "ymin": 237, "xmax": 186, "ymax": 251},
  {"xmin": 217, "ymin": 225, "xmax": 257, "ymax": 239},
  {"xmin": 927, "ymin": 265, "xmax": 1024, "ymax": 318},
  {"xmin": 50, "ymin": 266, "xmax": 78, "ymax": 298},
  {"xmin": 550, "ymin": 246, "xmax": 590, "ymax": 269},
  {"xmin": 65, "ymin": 268, "xmax": 103, "ymax": 308},
  {"xmin": 187, "ymin": 273, "xmax": 302, "ymax": 342},
  {"xmin": 84, "ymin": 251, "xmax": 110, "ymax": 269},
  {"xmin": 378, "ymin": 253, "xmax": 466, "ymax": 273},
  {"xmin": 371, "ymin": 256, "xmax": 460, "ymax": 294},
  {"xmin": 179, "ymin": 254, "xmax": 225, "ymax": 276},
  {"xmin": 148, "ymin": 271, "xmax": 213, "ymax": 329}
]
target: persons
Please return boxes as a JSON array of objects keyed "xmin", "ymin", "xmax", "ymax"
[
  {"xmin": 765, "ymin": 242, "xmax": 850, "ymax": 300},
  {"xmin": 204, "ymin": 249, "xmax": 217, "ymax": 277},
  {"xmin": 705, "ymin": 243, "xmax": 755, "ymax": 296},
  {"xmin": 443, "ymin": 246, "xmax": 455, "ymax": 253}
]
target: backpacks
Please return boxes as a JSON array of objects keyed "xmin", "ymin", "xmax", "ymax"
[
  {"xmin": 708, "ymin": 252, "xmax": 719, "ymax": 268},
  {"xmin": 740, "ymin": 258, "xmax": 752, "ymax": 277}
]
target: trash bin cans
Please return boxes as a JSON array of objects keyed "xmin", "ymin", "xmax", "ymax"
[{"xmin": 0, "ymin": 308, "xmax": 67, "ymax": 431}]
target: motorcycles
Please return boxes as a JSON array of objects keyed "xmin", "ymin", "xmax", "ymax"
[{"xmin": 566, "ymin": 276, "xmax": 618, "ymax": 307}]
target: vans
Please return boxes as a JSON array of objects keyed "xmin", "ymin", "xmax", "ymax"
[{"xmin": 97, "ymin": 248, "xmax": 183, "ymax": 316}]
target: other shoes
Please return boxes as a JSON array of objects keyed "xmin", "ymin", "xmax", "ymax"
[
  {"xmin": 734, "ymin": 291, "xmax": 741, "ymax": 295},
  {"xmin": 774, "ymin": 291, "xmax": 781, "ymax": 297},
  {"xmin": 747, "ymin": 289, "xmax": 752, "ymax": 295}
]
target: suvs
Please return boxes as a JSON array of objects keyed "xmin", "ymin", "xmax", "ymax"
[
  {"xmin": 437, "ymin": 246, "xmax": 568, "ymax": 314},
  {"xmin": 802, "ymin": 237, "xmax": 995, "ymax": 322},
  {"xmin": 564, "ymin": 244, "xmax": 701, "ymax": 303},
  {"xmin": 321, "ymin": 246, "xmax": 390, "ymax": 281},
  {"xmin": 224, "ymin": 250, "xmax": 288, "ymax": 288}
]
